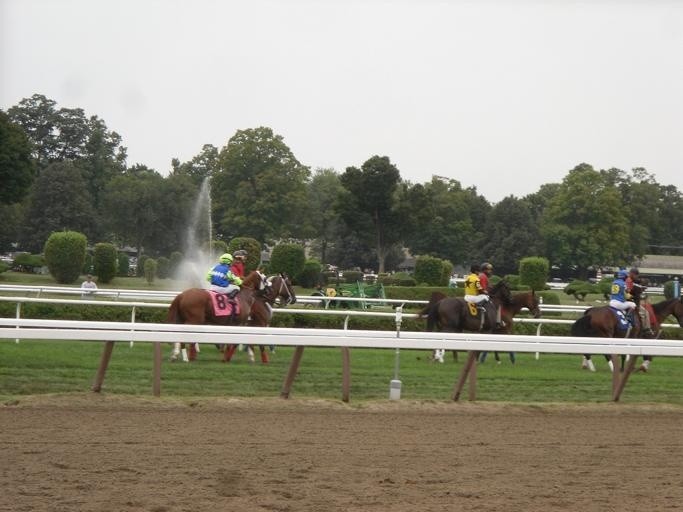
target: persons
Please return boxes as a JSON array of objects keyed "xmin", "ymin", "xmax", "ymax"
[
  {"xmin": 203, "ymin": 253, "xmax": 242, "ymax": 306},
  {"xmin": 79, "ymin": 274, "xmax": 97, "ymax": 300},
  {"xmin": 239, "ymin": 249, "xmax": 247, "ymax": 276},
  {"xmin": 478, "ymin": 262, "xmax": 493, "ymax": 295},
  {"xmin": 624, "ymin": 268, "xmax": 656, "ymax": 335},
  {"xmin": 229, "ymin": 250, "xmax": 242, "ymax": 278},
  {"xmin": 609, "ymin": 270, "xmax": 636, "ymax": 326},
  {"xmin": 461, "ymin": 262, "xmax": 502, "ymax": 332}
]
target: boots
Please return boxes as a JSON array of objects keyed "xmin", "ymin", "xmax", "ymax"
[
  {"xmin": 626, "ymin": 307, "xmax": 635, "ymax": 323},
  {"xmin": 228, "ymin": 289, "xmax": 239, "ymax": 305}
]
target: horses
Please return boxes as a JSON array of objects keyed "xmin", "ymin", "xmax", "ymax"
[
  {"xmin": 426, "ymin": 276, "xmax": 515, "ymax": 365},
  {"xmin": 214, "ymin": 270, "xmax": 297, "ymax": 355},
  {"xmin": 165, "ymin": 268, "xmax": 277, "ymax": 364},
  {"xmin": 570, "ymin": 274, "xmax": 653, "ymax": 373},
  {"xmin": 417, "ymin": 288, "xmax": 543, "ymax": 364},
  {"xmin": 581, "ymin": 295, "xmax": 683, "ymax": 372}
]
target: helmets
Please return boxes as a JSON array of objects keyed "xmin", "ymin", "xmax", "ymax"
[
  {"xmin": 480, "ymin": 263, "xmax": 494, "ymax": 273},
  {"xmin": 219, "ymin": 253, "xmax": 234, "ymax": 266},
  {"xmin": 233, "ymin": 250, "xmax": 248, "ymax": 263},
  {"xmin": 471, "ymin": 263, "xmax": 480, "ymax": 273},
  {"xmin": 617, "ymin": 271, "xmax": 627, "ymax": 281},
  {"xmin": 630, "ymin": 268, "xmax": 638, "ymax": 276}
]
